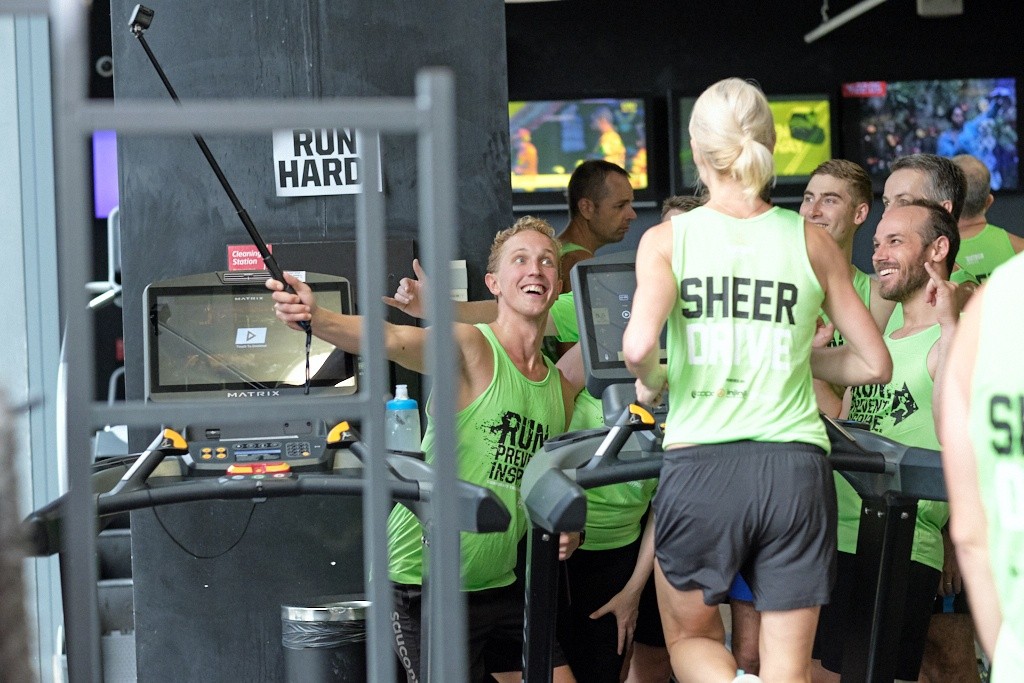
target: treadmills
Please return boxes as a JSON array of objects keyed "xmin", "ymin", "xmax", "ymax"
[
  {"xmin": 18, "ymin": 265, "xmax": 516, "ymax": 683},
  {"xmin": 519, "ymin": 248, "xmax": 968, "ymax": 683}
]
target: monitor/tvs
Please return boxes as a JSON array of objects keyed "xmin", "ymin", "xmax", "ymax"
[
  {"xmin": 573, "ymin": 260, "xmax": 670, "ymax": 370},
  {"xmin": 147, "ymin": 282, "xmax": 356, "ymax": 395},
  {"xmin": 843, "ymin": 74, "xmax": 1022, "ymax": 199},
  {"xmin": 507, "ymin": 92, "xmax": 660, "ymax": 213},
  {"xmin": 664, "ymin": 91, "xmax": 838, "ymax": 208}
]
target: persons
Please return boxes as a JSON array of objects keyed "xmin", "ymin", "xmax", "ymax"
[
  {"xmin": 265, "ymin": 215, "xmax": 588, "ymax": 683},
  {"xmin": 382, "ymin": 153, "xmax": 1024, "ymax": 683},
  {"xmin": 620, "ymin": 76, "xmax": 893, "ymax": 683},
  {"xmin": 583, "ymin": 107, "xmax": 648, "ymax": 189},
  {"xmin": 509, "ymin": 128, "xmax": 538, "ymax": 175},
  {"xmin": 861, "ymin": 86, "xmax": 1019, "ymax": 191}
]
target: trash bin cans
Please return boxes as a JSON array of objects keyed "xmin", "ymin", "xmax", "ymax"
[{"xmin": 280, "ymin": 598, "xmax": 371, "ymax": 683}]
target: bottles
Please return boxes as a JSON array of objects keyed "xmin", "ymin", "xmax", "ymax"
[{"xmin": 383, "ymin": 385, "xmax": 421, "ymax": 454}]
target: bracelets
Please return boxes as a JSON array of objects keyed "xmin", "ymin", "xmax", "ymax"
[{"xmin": 578, "ymin": 528, "xmax": 586, "ymax": 546}]
